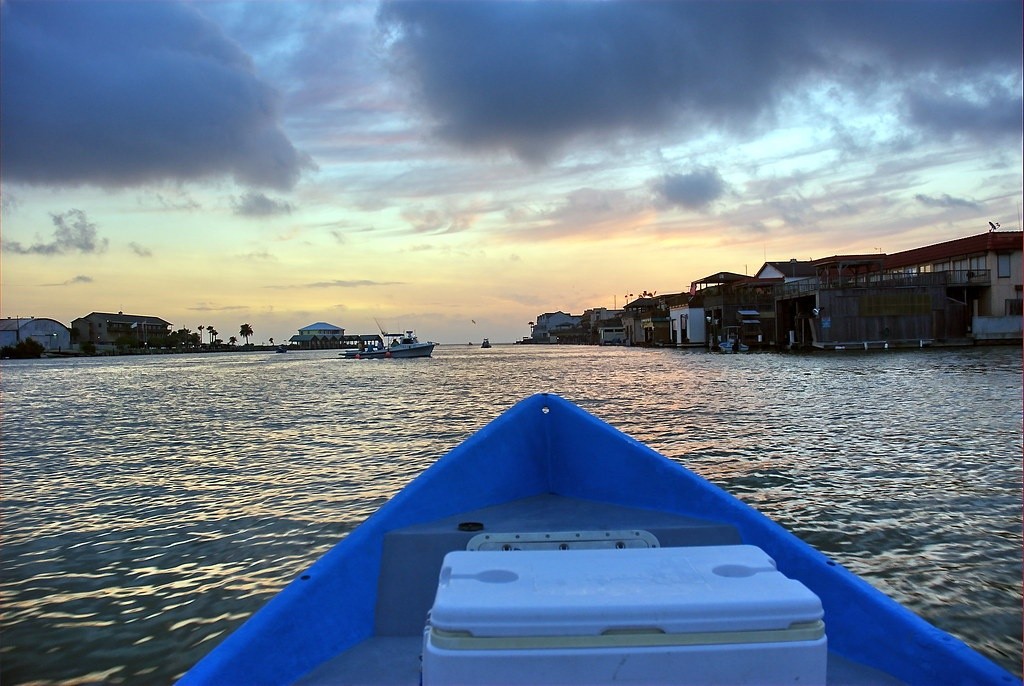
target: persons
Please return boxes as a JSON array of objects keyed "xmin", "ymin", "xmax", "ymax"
[
  {"xmin": 391, "ymin": 339, "xmax": 399, "ymax": 347},
  {"xmin": 377, "ymin": 340, "xmax": 382, "ymax": 350},
  {"xmin": 367, "ymin": 342, "xmax": 375, "ymax": 352},
  {"xmin": 358, "ymin": 342, "xmax": 365, "ymax": 352}
]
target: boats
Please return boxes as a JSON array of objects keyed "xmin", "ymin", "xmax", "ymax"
[
  {"xmin": 718, "ymin": 340, "xmax": 750, "ymax": 354},
  {"xmin": 276, "ymin": 347, "xmax": 288, "ymax": 354},
  {"xmin": 337, "ymin": 330, "xmax": 436, "ymax": 360},
  {"xmin": 481, "ymin": 338, "xmax": 493, "ymax": 348}
]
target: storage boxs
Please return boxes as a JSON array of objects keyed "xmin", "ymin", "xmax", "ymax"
[{"xmin": 421, "ymin": 545, "xmax": 828, "ymax": 686}]
